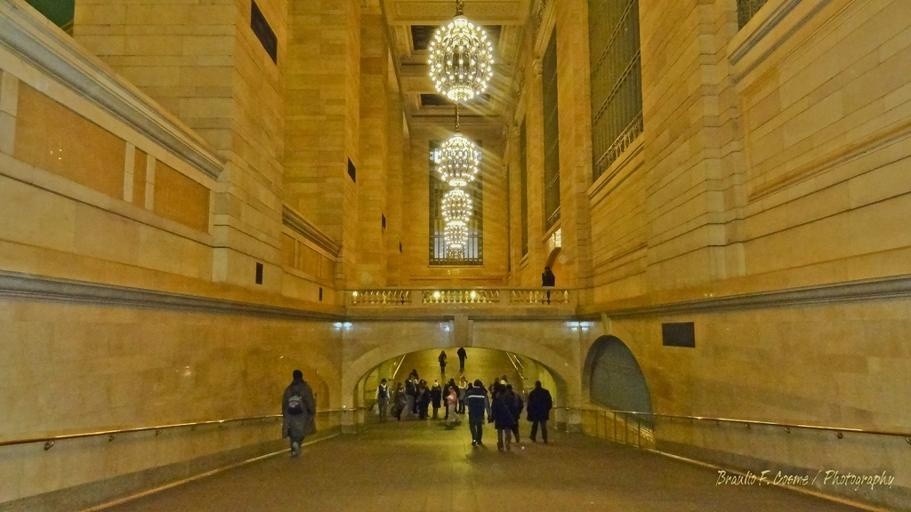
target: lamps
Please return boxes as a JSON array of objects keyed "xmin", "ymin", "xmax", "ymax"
[
  {"xmin": 434, "ymin": 102, "xmax": 481, "ymax": 188},
  {"xmin": 439, "ymin": 184, "xmax": 474, "ymax": 222},
  {"xmin": 443, "ymin": 221, "xmax": 469, "ymax": 250},
  {"xmin": 424, "ymin": 0, "xmax": 495, "ymax": 106}
]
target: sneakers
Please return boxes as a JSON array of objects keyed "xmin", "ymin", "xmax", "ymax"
[
  {"xmin": 471, "ymin": 439, "xmax": 482, "ymax": 447},
  {"xmin": 290, "ymin": 441, "xmax": 301, "ymax": 456}
]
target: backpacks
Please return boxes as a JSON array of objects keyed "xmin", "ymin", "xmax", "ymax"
[{"xmin": 286, "ymin": 382, "xmax": 307, "ymax": 415}]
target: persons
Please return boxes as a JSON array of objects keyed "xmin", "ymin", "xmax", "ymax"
[
  {"xmin": 456, "ymin": 348, "xmax": 467, "ymax": 372},
  {"xmin": 541, "ymin": 266, "xmax": 554, "ymax": 303},
  {"xmin": 375, "ymin": 367, "xmax": 553, "ymax": 450},
  {"xmin": 438, "ymin": 351, "xmax": 446, "ymax": 372},
  {"xmin": 279, "ymin": 371, "xmax": 316, "ymax": 458}
]
